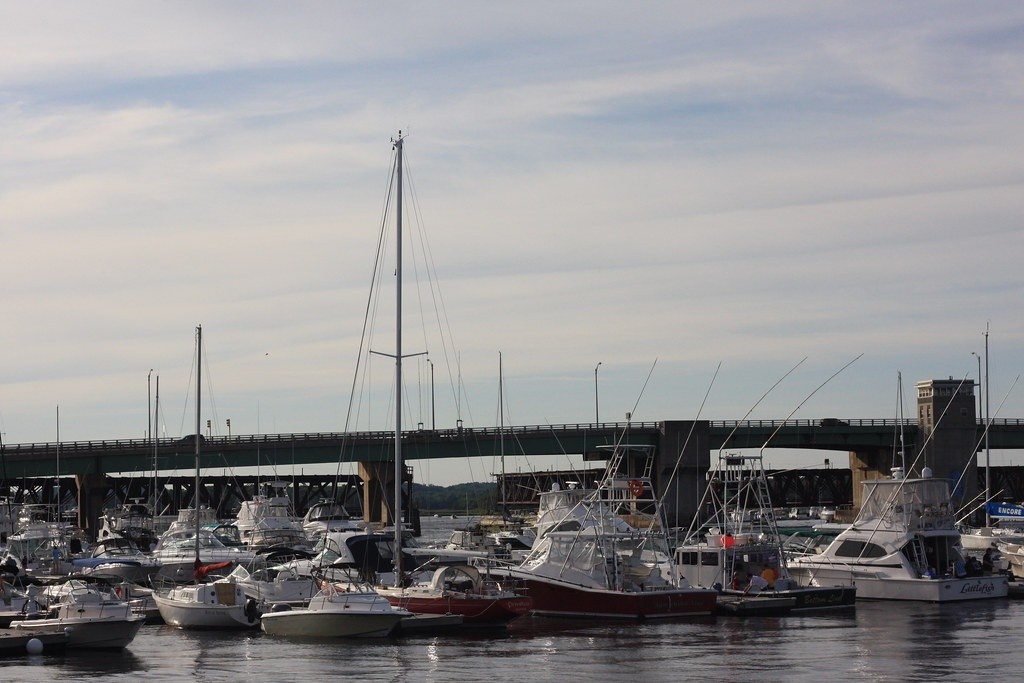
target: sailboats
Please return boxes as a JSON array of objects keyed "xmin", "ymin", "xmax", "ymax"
[
  {"xmin": 6, "ymin": 404, "xmax": 94, "ymax": 560},
  {"xmin": 954, "ymin": 319, "xmax": 1024, "ymax": 549},
  {"xmin": 488, "ymin": 350, "xmax": 534, "ymax": 564},
  {"xmin": 315, "ymin": 129, "xmax": 535, "ymax": 611},
  {"xmin": 124, "ymin": 366, "xmax": 175, "ymax": 536}
]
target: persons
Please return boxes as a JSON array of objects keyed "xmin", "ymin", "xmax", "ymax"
[
  {"xmin": 983, "ymin": 548, "xmax": 992, "ymax": 571},
  {"xmin": 70, "ymin": 535, "xmax": 82, "ymax": 554},
  {"xmin": 744, "ymin": 573, "xmax": 772, "ymax": 592},
  {"xmin": 52, "ymin": 545, "xmax": 60, "ymax": 575},
  {"xmin": 761, "ymin": 564, "xmax": 775, "ymax": 587},
  {"xmin": 6, "ymin": 556, "xmax": 16, "ymax": 574}
]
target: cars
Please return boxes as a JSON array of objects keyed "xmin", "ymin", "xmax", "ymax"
[
  {"xmin": 819, "ymin": 418, "xmax": 848, "ymax": 427},
  {"xmin": 731, "ymin": 508, "xmax": 836, "ymax": 524},
  {"xmin": 172, "ymin": 434, "xmax": 205, "ymax": 444}
]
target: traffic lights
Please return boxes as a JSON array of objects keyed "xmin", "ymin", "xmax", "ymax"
[
  {"xmin": 226, "ymin": 418, "xmax": 230, "ymax": 427},
  {"xmin": 207, "ymin": 419, "xmax": 211, "ymax": 428}
]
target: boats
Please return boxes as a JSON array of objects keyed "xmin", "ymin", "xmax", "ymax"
[
  {"xmin": 226, "ymin": 526, "xmax": 489, "ymax": 605},
  {"xmin": 0, "ymin": 588, "xmax": 148, "ymax": 651},
  {"xmin": 478, "ymin": 354, "xmax": 719, "ymax": 618},
  {"xmin": 301, "ymin": 496, "xmax": 415, "ymax": 548},
  {"xmin": 445, "ymin": 491, "xmax": 488, "ymax": 551},
  {"xmin": 154, "ymin": 505, "xmax": 231, "ymax": 548},
  {"xmin": 451, "ymin": 513, "xmax": 456, "ymax": 518},
  {"xmin": 259, "ymin": 582, "xmax": 464, "ymax": 642},
  {"xmin": 785, "ymin": 373, "xmax": 1010, "ymax": 603},
  {"xmin": 674, "ymin": 354, "xmax": 865, "ymax": 608},
  {"xmin": 716, "ymin": 574, "xmax": 797, "ymax": 614},
  {"xmin": 0, "ymin": 496, "xmax": 29, "ymax": 543},
  {"xmin": 992, "ymin": 536, "xmax": 1024, "ymax": 579},
  {"xmin": 152, "ymin": 562, "xmax": 271, "ymax": 628},
  {"xmin": 766, "ymin": 516, "xmax": 848, "ymax": 561},
  {"xmin": 44, "ymin": 575, "xmax": 112, "ymax": 602},
  {"xmin": 96, "ymin": 500, "xmax": 160, "ymax": 555},
  {"xmin": 55, "ymin": 573, "xmax": 162, "ymax": 623},
  {"xmin": 70, "ymin": 545, "xmax": 309, "ymax": 585},
  {"xmin": 0, "ymin": 550, "xmax": 51, "ymax": 626},
  {"xmin": 433, "ymin": 513, "xmax": 441, "ymax": 517},
  {"xmin": 230, "ymin": 479, "xmax": 305, "ymax": 552}
]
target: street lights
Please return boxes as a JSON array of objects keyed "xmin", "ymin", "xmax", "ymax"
[
  {"xmin": 972, "ymin": 351, "xmax": 981, "ymax": 425},
  {"xmin": 594, "ymin": 361, "xmax": 603, "ymax": 430},
  {"xmin": 147, "ymin": 368, "xmax": 152, "ymax": 449},
  {"xmin": 427, "ymin": 359, "xmax": 434, "ymax": 437}
]
